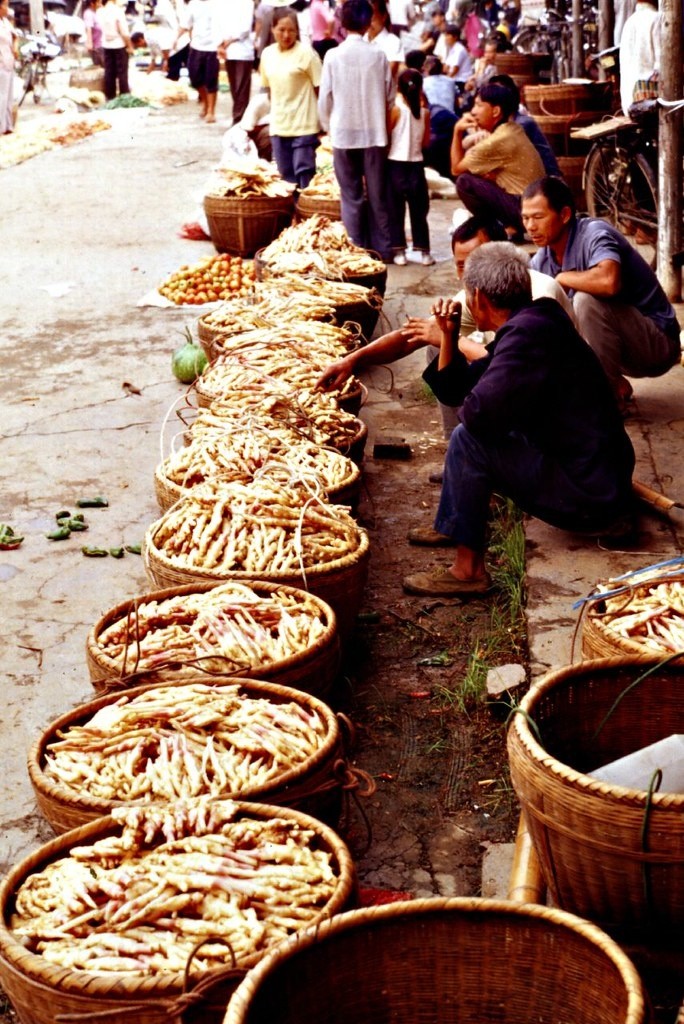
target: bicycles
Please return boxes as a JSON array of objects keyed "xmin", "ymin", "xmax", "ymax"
[
  {"xmin": 14, "ymin": 34, "xmax": 49, "ymax": 107},
  {"xmin": 580, "ymin": 96, "xmax": 659, "ymax": 270},
  {"xmin": 505, "ymin": 6, "xmax": 621, "ymax": 83}
]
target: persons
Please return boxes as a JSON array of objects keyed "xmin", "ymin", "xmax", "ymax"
[
  {"xmin": 359, "ymin": 1, "xmax": 407, "ymax": 63},
  {"xmin": 448, "ymin": 73, "xmax": 569, "ymax": 244},
  {"xmin": 387, "ymin": 69, "xmax": 435, "ymax": 266},
  {"xmin": 0, "ymin": 0, "xmax": 19, "ymax": 135},
  {"xmin": 397, "ymin": 241, "xmax": 643, "ymax": 595},
  {"xmin": 82, "ymin": 0, "xmax": 193, "ymax": 98},
  {"xmin": 316, "ymin": 0, "xmax": 395, "ymax": 264},
  {"xmin": 619, "ymin": 0, "xmax": 664, "ymax": 115},
  {"xmin": 521, "ymin": 176, "xmax": 681, "ymax": 421},
  {"xmin": 172, "ymin": 0, "xmax": 346, "ymax": 195},
  {"xmin": 401, "ymin": 0, "xmax": 596, "ymax": 187},
  {"xmin": 312, "ymin": 215, "xmax": 582, "ymax": 483}
]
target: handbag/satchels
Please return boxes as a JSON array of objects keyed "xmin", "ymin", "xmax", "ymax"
[{"xmin": 632, "ymin": 73, "xmax": 658, "ymax": 102}]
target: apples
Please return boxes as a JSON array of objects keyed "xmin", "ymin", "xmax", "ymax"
[{"xmin": 159, "ymin": 253, "xmax": 255, "ymax": 305}]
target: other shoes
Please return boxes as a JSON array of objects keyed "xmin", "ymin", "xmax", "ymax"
[
  {"xmin": 616, "ymin": 218, "xmax": 658, "ymax": 246},
  {"xmin": 405, "ymin": 248, "xmax": 433, "ymax": 265},
  {"xmin": 485, "ymin": 496, "xmax": 505, "ymax": 518},
  {"xmin": 392, "ymin": 251, "xmax": 406, "ymax": 265},
  {"xmin": 428, "ymin": 471, "xmax": 444, "ymax": 482},
  {"xmin": 614, "ymin": 377, "xmax": 633, "ymax": 418}
]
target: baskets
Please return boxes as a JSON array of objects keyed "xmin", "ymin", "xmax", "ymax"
[
  {"xmin": 296, "ymin": 189, "xmax": 341, "ymax": 222},
  {"xmin": 507, "ymin": 651, "xmax": 683, "ymax": 965},
  {"xmin": 578, "ymin": 587, "xmax": 647, "ymax": 662},
  {"xmin": 0, "ymin": 246, "xmax": 388, "ymax": 1024},
  {"xmin": 493, "ymin": 52, "xmax": 605, "ymax": 214},
  {"xmin": 204, "ymin": 194, "xmax": 295, "ymax": 259},
  {"xmin": 221, "ymin": 897, "xmax": 644, "ymax": 1024}
]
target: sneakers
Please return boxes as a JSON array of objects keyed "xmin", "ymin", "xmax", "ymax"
[
  {"xmin": 402, "ymin": 564, "xmax": 491, "ymax": 597},
  {"xmin": 407, "ymin": 525, "xmax": 457, "ymax": 545}
]
